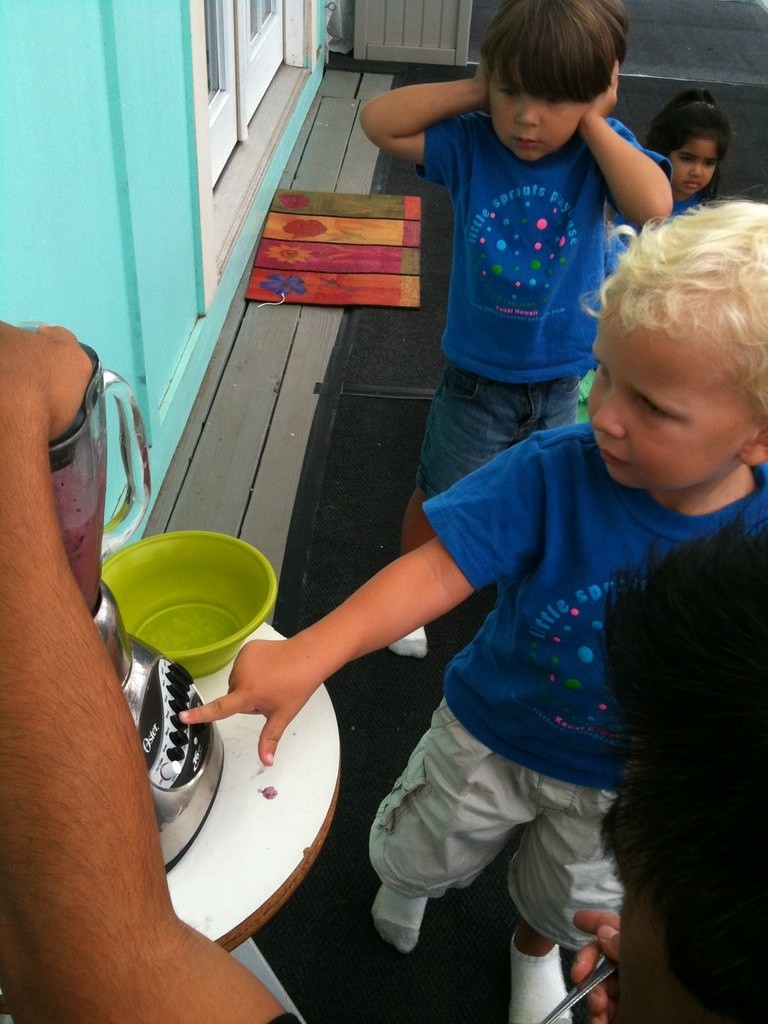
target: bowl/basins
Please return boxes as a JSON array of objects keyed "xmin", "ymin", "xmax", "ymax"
[{"xmin": 102, "ymin": 528, "xmax": 279, "ymax": 677}]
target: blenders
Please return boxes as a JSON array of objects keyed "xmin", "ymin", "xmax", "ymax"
[{"xmin": 10, "ymin": 318, "xmax": 226, "ymax": 874}]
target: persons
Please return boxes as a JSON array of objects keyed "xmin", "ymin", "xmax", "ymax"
[
  {"xmin": 0, "ymin": 323, "xmax": 768, "ymax": 1024},
  {"xmin": 359, "ymin": 0, "xmax": 677, "ymax": 665},
  {"xmin": 178, "ymin": 201, "xmax": 768, "ymax": 1019},
  {"xmin": 607, "ymin": 86, "xmax": 731, "ymax": 283}
]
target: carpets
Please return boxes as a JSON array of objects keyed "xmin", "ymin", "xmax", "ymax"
[{"xmin": 244, "ymin": 186, "xmax": 424, "ymax": 312}]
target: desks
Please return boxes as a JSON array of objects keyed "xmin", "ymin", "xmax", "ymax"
[{"xmin": 109, "ymin": 549, "xmax": 344, "ymax": 1023}]
